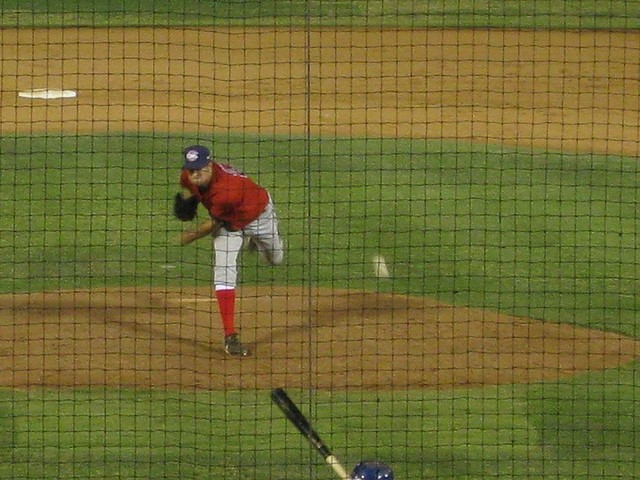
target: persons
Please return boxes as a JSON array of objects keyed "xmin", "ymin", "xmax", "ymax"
[
  {"xmin": 350, "ymin": 459, "xmax": 395, "ymax": 480},
  {"xmin": 172, "ymin": 144, "xmax": 285, "ymax": 358}
]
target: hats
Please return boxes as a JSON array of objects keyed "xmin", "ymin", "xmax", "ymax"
[{"xmin": 183, "ymin": 144, "xmax": 212, "ymax": 170}]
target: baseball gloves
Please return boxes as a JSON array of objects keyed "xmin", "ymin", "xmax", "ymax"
[{"xmin": 174, "ymin": 193, "xmax": 198, "ymax": 221}]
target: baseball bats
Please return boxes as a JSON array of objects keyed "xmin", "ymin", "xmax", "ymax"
[{"xmin": 271, "ymin": 388, "xmax": 351, "ymax": 480}]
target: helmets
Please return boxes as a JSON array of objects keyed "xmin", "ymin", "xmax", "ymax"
[{"xmin": 351, "ymin": 461, "xmax": 392, "ymax": 479}]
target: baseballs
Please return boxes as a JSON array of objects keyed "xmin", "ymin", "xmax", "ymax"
[{"xmin": 372, "ymin": 255, "xmax": 390, "ymax": 278}]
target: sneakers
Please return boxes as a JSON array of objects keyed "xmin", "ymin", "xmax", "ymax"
[{"xmin": 224, "ymin": 332, "xmax": 248, "ymax": 356}]
